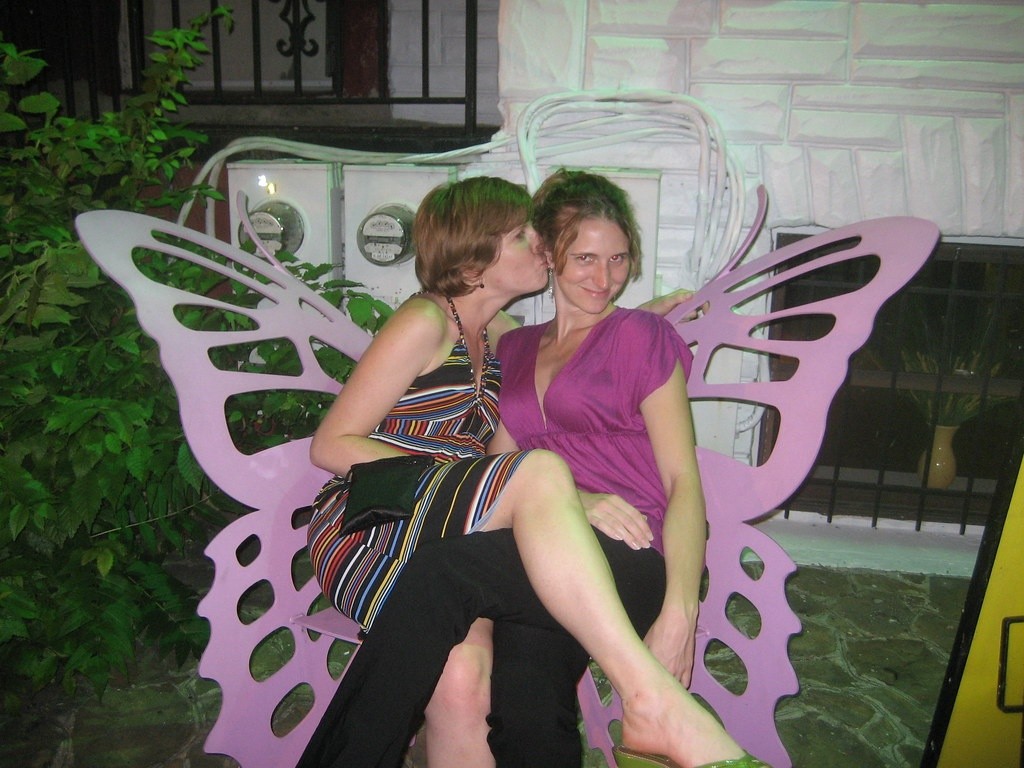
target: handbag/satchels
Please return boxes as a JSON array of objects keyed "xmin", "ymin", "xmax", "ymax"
[{"xmin": 337, "ymin": 455, "xmax": 434, "ymax": 538}]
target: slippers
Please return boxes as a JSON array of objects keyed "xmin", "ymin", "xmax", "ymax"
[{"xmin": 611, "ymin": 741, "xmax": 776, "ymax": 768}]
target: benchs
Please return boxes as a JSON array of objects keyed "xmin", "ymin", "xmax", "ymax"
[{"xmin": 289, "ymin": 607, "xmax": 362, "ymax": 648}]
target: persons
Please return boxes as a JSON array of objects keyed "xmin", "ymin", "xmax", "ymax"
[
  {"xmin": 295, "ymin": 168, "xmax": 706, "ymax": 768},
  {"xmin": 306, "ymin": 176, "xmax": 777, "ymax": 768}
]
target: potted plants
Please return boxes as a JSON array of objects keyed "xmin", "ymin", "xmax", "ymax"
[{"xmin": 901, "ymin": 346, "xmax": 1012, "ymax": 490}]
